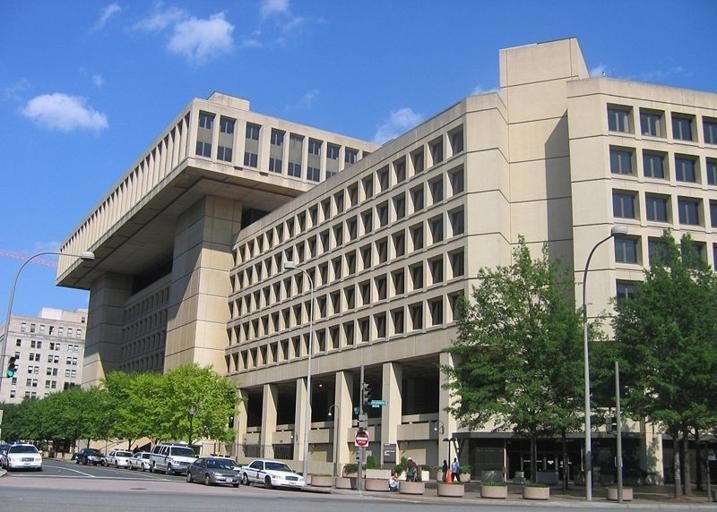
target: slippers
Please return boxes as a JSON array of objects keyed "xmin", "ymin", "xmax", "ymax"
[{"xmin": 407, "ymin": 469, "xmax": 412, "ymax": 476}]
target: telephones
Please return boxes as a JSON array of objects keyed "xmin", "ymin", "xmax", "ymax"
[{"xmin": 371, "ymin": 400, "xmax": 388, "ymax": 408}]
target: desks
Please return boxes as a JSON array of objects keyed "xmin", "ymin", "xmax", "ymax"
[
  {"xmin": 189, "ymin": 406, "xmax": 195, "ymax": 444},
  {"xmin": 328, "ymin": 405, "xmax": 339, "ymax": 477},
  {"xmin": 1, "ymin": 251, "xmax": 95, "ymax": 380},
  {"xmin": 285, "ymin": 261, "xmax": 314, "ymax": 476},
  {"xmin": 443, "ymin": 438, "xmax": 456, "ymax": 468},
  {"xmin": 583, "ymin": 226, "xmax": 626, "ymax": 500}
]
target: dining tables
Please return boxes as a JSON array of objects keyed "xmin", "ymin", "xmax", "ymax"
[
  {"xmin": 481, "ymin": 480, "xmax": 507, "ymax": 499},
  {"xmin": 434, "ymin": 465, "xmax": 443, "ymax": 482},
  {"xmin": 457, "ymin": 464, "xmax": 473, "ymax": 483},
  {"xmin": 418, "ymin": 464, "xmax": 433, "ymax": 481},
  {"xmin": 335, "ymin": 463, "xmax": 359, "ymax": 489},
  {"xmin": 607, "ymin": 482, "xmax": 634, "ymax": 500},
  {"xmin": 521, "ymin": 478, "xmax": 550, "ymax": 500}
]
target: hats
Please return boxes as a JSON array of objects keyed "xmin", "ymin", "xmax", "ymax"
[
  {"xmin": 242, "ymin": 460, "xmax": 306, "ymax": 489},
  {"xmin": 0, "ymin": 444, "xmax": 42, "ymax": 470},
  {"xmin": 104, "ymin": 449, "xmax": 134, "ymax": 469},
  {"xmin": 129, "ymin": 451, "xmax": 151, "ymax": 471},
  {"xmin": 186, "ymin": 457, "xmax": 241, "ymax": 487},
  {"xmin": 74, "ymin": 449, "xmax": 105, "ymax": 464}
]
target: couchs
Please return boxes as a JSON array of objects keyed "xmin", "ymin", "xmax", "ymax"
[
  {"xmin": 7, "ymin": 356, "xmax": 17, "ymax": 379},
  {"xmin": 363, "ymin": 383, "xmax": 371, "ymax": 406}
]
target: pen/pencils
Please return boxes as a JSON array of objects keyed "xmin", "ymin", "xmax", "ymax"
[{"xmin": 150, "ymin": 441, "xmax": 198, "ymax": 474}]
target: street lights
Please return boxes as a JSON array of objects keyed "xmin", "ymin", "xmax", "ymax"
[{"xmin": 456, "ymin": 468, "xmax": 461, "ymax": 474}]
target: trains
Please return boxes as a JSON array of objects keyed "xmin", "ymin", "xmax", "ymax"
[{"xmin": 354, "ymin": 430, "xmax": 369, "ymax": 447}]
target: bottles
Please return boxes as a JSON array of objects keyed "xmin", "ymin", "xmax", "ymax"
[{"xmin": 408, "ymin": 457, "xmax": 412, "ymax": 459}]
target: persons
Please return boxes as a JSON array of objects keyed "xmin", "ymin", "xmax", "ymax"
[
  {"xmin": 445, "ymin": 466, "xmax": 453, "ymax": 484},
  {"xmin": 442, "ymin": 460, "xmax": 447, "ymax": 483},
  {"xmin": 389, "ymin": 451, "xmax": 424, "ymax": 491},
  {"xmin": 451, "ymin": 457, "xmax": 462, "ymax": 482}
]
